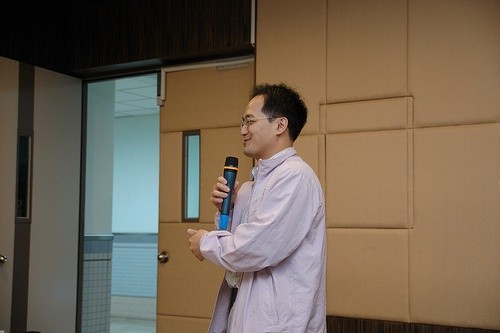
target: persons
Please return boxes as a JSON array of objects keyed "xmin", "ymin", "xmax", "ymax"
[{"xmin": 186, "ymin": 84, "xmax": 328, "ymax": 333}]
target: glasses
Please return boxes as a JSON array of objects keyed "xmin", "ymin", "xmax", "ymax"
[{"xmin": 239, "ymin": 112, "xmax": 275, "ymax": 127}]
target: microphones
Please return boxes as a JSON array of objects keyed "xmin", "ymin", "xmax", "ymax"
[{"xmin": 220, "ymin": 156, "xmax": 239, "ymax": 231}]
